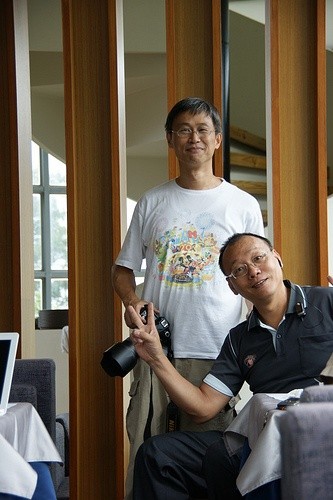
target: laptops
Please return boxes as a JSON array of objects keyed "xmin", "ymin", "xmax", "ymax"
[{"xmin": 0, "ymin": 332, "xmax": 20, "ymax": 416}]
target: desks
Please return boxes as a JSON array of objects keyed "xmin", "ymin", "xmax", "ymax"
[
  {"xmin": 0, "ymin": 402, "xmax": 64, "ymax": 500},
  {"xmin": 221, "ymin": 393, "xmax": 301, "ymax": 500}
]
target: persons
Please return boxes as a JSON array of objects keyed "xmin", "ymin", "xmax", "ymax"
[
  {"xmin": 128, "ymin": 233, "xmax": 333, "ymax": 500},
  {"xmin": 111, "ymin": 98, "xmax": 265, "ymax": 500}
]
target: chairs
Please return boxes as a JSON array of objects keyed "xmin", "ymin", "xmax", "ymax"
[
  {"xmin": 9, "ymin": 358, "xmax": 70, "ymax": 500},
  {"xmin": 281, "ymin": 385, "xmax": 333, "ymax": 500}
]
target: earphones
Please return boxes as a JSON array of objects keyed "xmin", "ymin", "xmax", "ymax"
[{"xmin": 278, "ymin": 259, "xmax": 281, "ymax": 267}]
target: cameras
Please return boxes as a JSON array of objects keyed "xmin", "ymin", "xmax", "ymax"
[{"xmin": 101, "ymin": 305, "xmax": 172, "ymax": 378}]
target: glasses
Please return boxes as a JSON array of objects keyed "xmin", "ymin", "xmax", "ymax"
[
  {"xmin": 167, "ymin": 127, "xmax": 217, "ymax": 137},
  {"xmin": 227, "ymin": 255, "xmax": 267, "ymax": 279}
]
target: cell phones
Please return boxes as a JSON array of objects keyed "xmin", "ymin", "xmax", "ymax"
[{"xmin": 278, "ymin": 398, "xmax": 300, "ymax": 410}]
